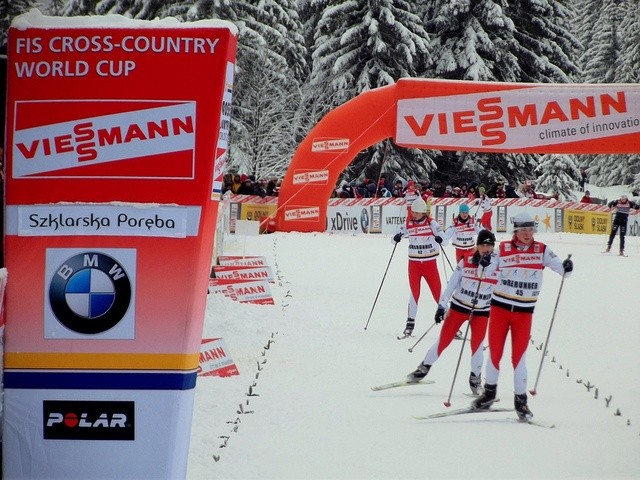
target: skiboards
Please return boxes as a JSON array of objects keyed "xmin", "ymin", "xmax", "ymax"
[
  {"xmin": 411, "ymin": 397, "xmax": 556, "ymax": 429},
  {"xmin": 370, "ymin": 380, "xmax": 500, "ymax": 402},
  {"xmin": 397, "ymin": 329, "xmax": 463, "ymax": 340}
]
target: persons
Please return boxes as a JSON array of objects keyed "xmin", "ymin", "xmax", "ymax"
[
  {"xmin": 475, "ymin": 186, "xmax": 492, "ymax": 232},
  {"xmin": 221, "ymin": 173, "xmax": 280, "ymax": 197},
  {"xmin": 580, "ymin": 190, "xmax": 592, "ymax": 203},
  {"xmin": 393, "ymin": 195, "xmax": 449, "ymax": 334},
  {"xmin": 408, "ymin": 229, "xmax": 499, "ymax": 397},
  {"xmin": 331, "ymin": 178, "xmax": 537, "ymax": 198},
  {"xmin": 602, "ymin": 194, "xmax": 640, "ymax": 257},
  {"xmin": 443, "ymin": 204, "xmax": 486, "ymax": 264},
  {"xmin": 472, "ymin": 214, "xmax": 573, "ymax": 416}
]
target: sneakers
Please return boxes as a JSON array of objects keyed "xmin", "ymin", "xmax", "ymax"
[
  {"xmin": 455, "ymin": 330, "xmax": 462, "ymax": 339},
  {"xmin": 515, "ymin": 394, "xmax": 533, "ymax": 419},
  {"xmin": 408, "ymin": 362, "xmax": 431, "ymax": 381},
  {"xmin": 472, "ymin": 384, "xmax": 497, "ymax": 408},
  {"xmin": 469, "ymin": 372, "xmax": 484, "ymax": 396},
  {"xmin": 404, "ymin": 317, "xmax": 414, "ymax": 335}
]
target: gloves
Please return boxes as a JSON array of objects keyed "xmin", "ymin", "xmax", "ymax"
[
  {"xmin": 480, "ymin": 251, "xmax": 491, "ymax": 267},
  {"xmin": 562, "ymin": 260, "xmax": 573, "ymax": 272},
  {"xmin": 435, "ymin": 304, "xmax": 444, "ymax": 323},
  {"xmin": 435, "ymin": 236, "xmax": 443, "ymax": 243},
  {"xmin": 393, "ymin": 234, "xmax": 401, "ymax": 242}
]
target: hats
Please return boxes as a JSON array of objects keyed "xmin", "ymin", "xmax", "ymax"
[
  {"xmin": 459, "ymin": 204, "xmax": 469, "ymax": 212},
  {"xmin": 513, "ymin": 213, "xmax": 537, "ymax": 232},
  {"xmin": 477, "ymin": 230, "xmax": 494, "ymax": 247},
  {"xmin": 411, "ymin": 196, "xmax": 427, "ymax": 213},
  {"xmin": 479, "ymin": 187, "xmax": 485, "ymax": 192}
]
